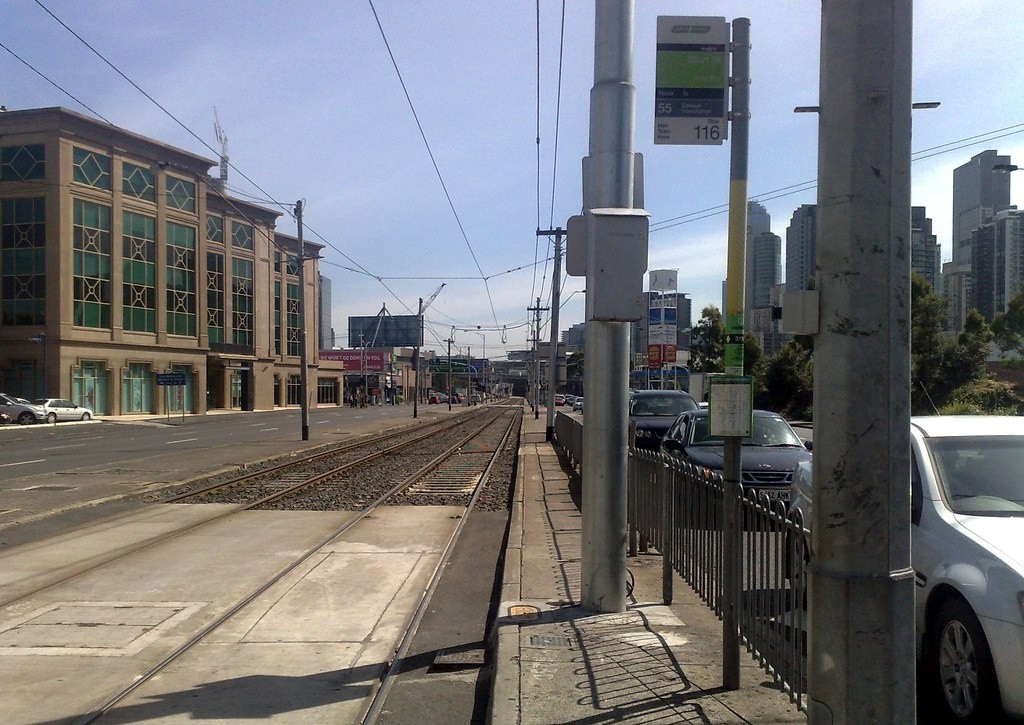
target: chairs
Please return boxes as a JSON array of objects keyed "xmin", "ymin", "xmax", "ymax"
[{"xmin": 633, "ymin": 402, "xmax": 647, "ymax": 413}]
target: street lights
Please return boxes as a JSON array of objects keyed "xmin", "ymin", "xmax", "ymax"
[
  {"xmin": 534, "ymin": 289, "xmax": 587, "ymax": 420},
  {"xmin": 465, "ymin": 329, "xmax": 486, "ymax": 389},
  {"xmin": 362, "ymin": 340, "xmax": 371, "ymax": 397}
]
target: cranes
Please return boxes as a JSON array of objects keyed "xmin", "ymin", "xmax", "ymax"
[{"xmin": 212, "ymin": 104, "xmax": 229, "ymax": 181}]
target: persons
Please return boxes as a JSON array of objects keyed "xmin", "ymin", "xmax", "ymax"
[
  {"xmin": 350, "ymin": 395, "xmax": 354, "ymax": 407},
  {"xmin": 351, "ymin": 393, "xmax": 358, "ymax": 407}
]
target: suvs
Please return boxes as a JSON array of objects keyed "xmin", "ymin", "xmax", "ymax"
[{"xmin": 628, "ymin": 388, "xmax": 702, "ymax": 450}]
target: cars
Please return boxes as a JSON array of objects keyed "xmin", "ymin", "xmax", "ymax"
[
  {"xmin": 0, "ymin": 392, "xmax": 95, "ymax": 426},
  {"xmin": 660, "ymin": 402, "xmax": 813, "ymax": 503},
  {"xmin": 552, "ymin": 393, "xmax": 585, "ymax": 412},
  {"xmin": 785, "ymin": 416, "xmax": 1024, "ymax": 725},
  {"xmin": 429, "ymin": 392, "xmax": 482, "ymax": 404}
]
